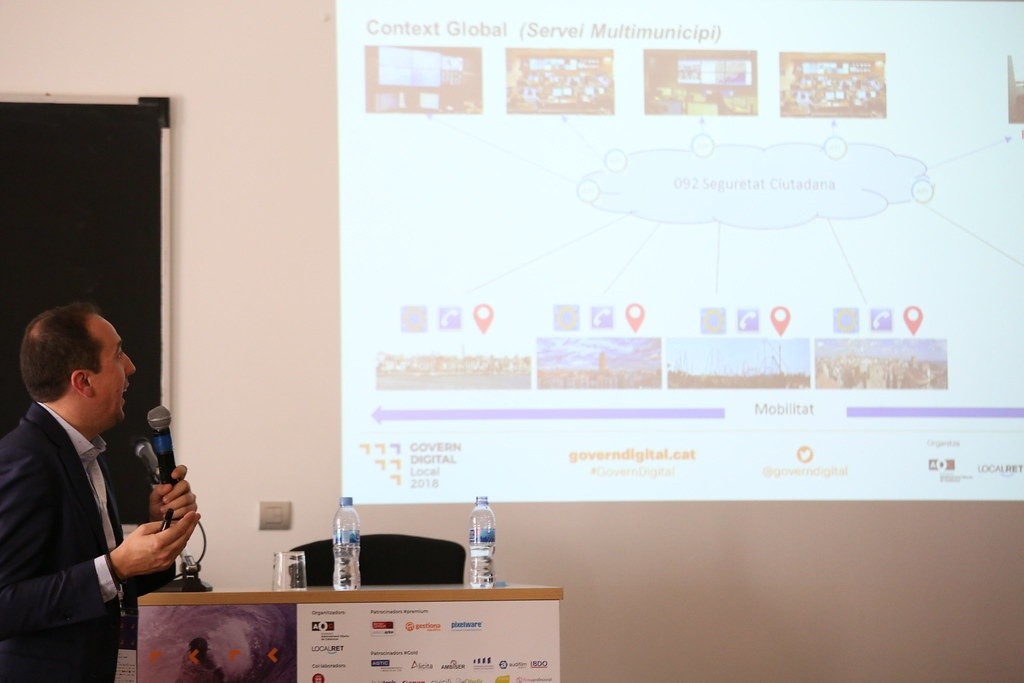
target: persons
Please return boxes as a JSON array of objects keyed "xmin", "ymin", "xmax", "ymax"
[{"xmin": 0, "ymin": 302, "xmax": 201, "ymax": 682}]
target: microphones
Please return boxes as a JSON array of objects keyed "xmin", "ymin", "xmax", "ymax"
[
  {"xmin": 130, "ymin": 437, "xmax": 162, "ymax": 484},
  {"xmin": 148, "ymin": 406, "xmax": 179, "ymax": 486}
]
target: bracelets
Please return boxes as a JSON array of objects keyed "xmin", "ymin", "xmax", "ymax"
[{"xmin": 105, "ymin": 550, "xmax": 127, "ymax": 584}]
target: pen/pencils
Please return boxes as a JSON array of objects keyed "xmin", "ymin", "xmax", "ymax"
[{"xmin": 161, "ymin": 508, "xmax": 174, "ymax": 532}]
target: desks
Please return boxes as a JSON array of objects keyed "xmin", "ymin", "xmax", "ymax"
[{"xmin": 137, "ymin": 584, "xmax": 564, "ymax": 683}]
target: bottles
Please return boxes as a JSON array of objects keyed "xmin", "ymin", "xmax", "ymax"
[
  {"xmin": 332, "ymin": 497, "xmax": 361, "ymax": 591},
  {"xmin": 468, "ymin": 496, "xmax": 496, "ymax": 588}
]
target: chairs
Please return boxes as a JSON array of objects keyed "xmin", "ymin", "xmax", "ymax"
[{"xmin": 288, "ymin": 534, "xmax": 466, "ymax": 587}]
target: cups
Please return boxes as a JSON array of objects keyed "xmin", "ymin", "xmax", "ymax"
[{"xmin": 270, "ymin": 551, "xmax": 306, "ymax": 591}]
